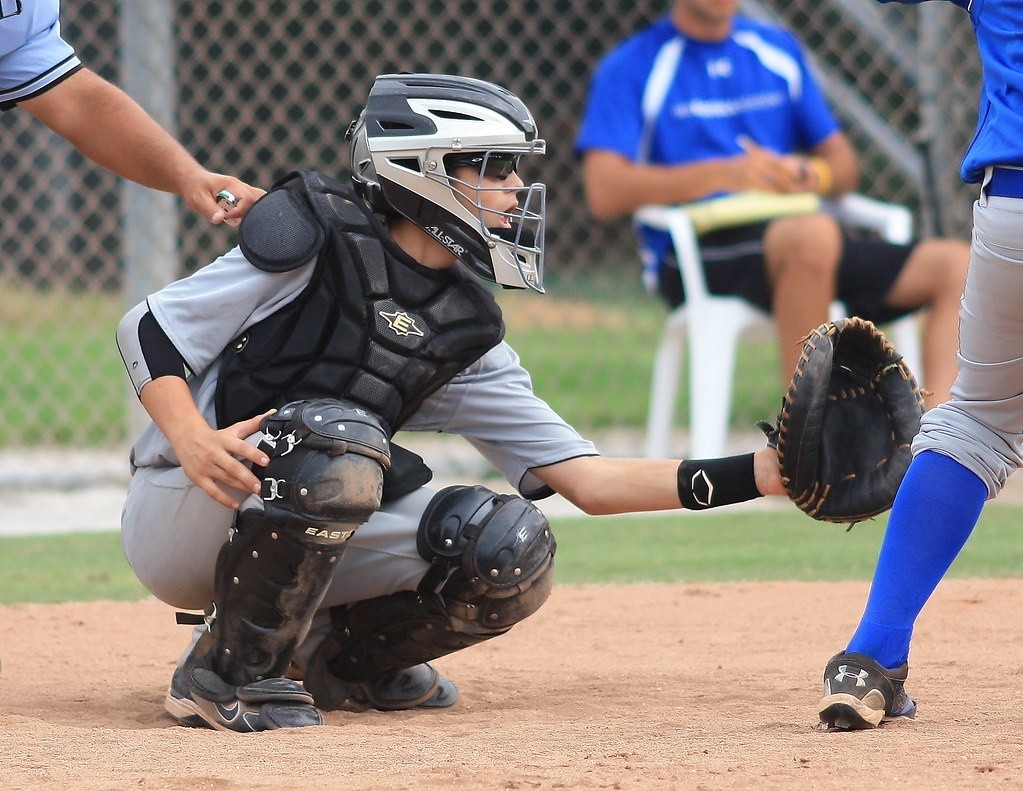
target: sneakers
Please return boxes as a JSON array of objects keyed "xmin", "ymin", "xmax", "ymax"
[
  {"xmin": 163, "ymin": 626, "xmax": 324, "ymax": 736},
  {"xmin": 305, "ymin": 643, "xmax": 457, "ymax": 708},
  {"xmin": 819, "ymin": 649, "xmax": 917, "ymax": 729}
]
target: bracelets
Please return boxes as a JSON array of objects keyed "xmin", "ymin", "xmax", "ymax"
[{"xmin": 811, "ymin": 158, "xmax": 832, "ymax": 195}]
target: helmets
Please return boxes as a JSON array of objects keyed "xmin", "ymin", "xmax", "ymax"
[{"xmin": 349, "ymin": 73, "xmax": 550, "ymax": 293}]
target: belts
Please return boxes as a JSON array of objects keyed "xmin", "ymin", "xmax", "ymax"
[{"xmin": 983, "ymin": 167, "xmax": 1023, "ymax": 198}]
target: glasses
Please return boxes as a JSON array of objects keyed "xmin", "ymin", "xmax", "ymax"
[{"xmin": 441, "ymin": 151, "xmax": 524, "ymax": 181}]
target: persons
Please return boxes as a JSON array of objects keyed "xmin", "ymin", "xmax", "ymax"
[
  {"xmin": 0, "ymin": 0, "xmax": 267, "ymax": 222},
  {"xmin": 113, "ymin": 73, "xmax": 790, "ymax": 732},
  {"xmin": 572, "ymin": 0, "xmax": 974, "ymax": 413},
  {"xmin": 817, "ymin": 0, "xmax": 1023, "ymax": 725}
]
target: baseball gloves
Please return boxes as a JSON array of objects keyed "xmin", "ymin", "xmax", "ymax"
[{"xmin": 751, "ymin": 317, "xmax": 935, "ymax": 534}]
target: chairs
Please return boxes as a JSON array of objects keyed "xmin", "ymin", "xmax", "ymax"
[{"xmin": 633, "ymin": 192, "xmax": 921, "ymax": 461}]
